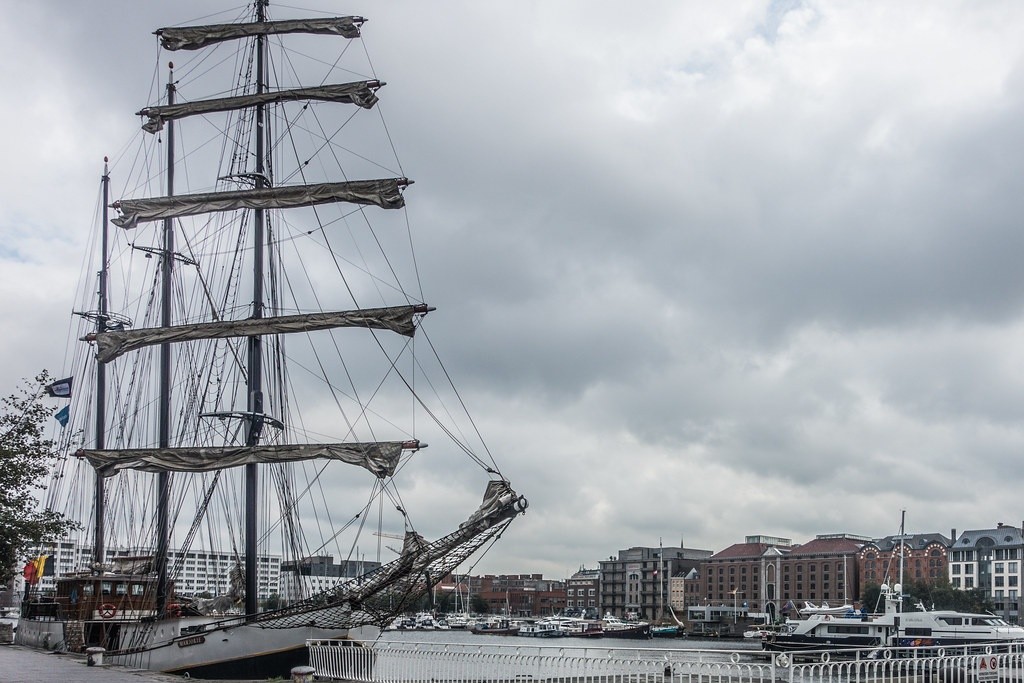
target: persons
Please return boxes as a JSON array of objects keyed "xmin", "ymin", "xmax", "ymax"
[{"xmin": 860, "ymin": 604, "xmax": 868, "ymax": 622}]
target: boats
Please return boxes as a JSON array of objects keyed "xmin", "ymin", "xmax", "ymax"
[{"xmin": 465, "ymin": 616, "xmax": 686, "ymax": 638}]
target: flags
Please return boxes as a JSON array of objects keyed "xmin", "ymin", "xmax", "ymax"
[
  {"xmin": 22, "ymin": 556, "xmax": 53, "ymax": 585},
  {"xmin": 44, "ymin": 376, "xmax": 73, "ymax": 398},
  {"xmin": 54, "ymin": 404, "xmax": 70, "ymax": 427},
  {"xmin": 779, "ymin": 600, "xmax": 793, "ymax": 613}
]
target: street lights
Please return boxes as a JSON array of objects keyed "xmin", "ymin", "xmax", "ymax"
[{"xmin": 732, "ymin": 591, "xmax": 737, "ymax": 625}]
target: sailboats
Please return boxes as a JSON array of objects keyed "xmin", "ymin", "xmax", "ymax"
[
  {"xmin": 760, "ymin": 510, "xmax": 1024, "ymax": 656},
  {"xmin": 382, "ymin": 566, "xmax": 477, "ymax": 631},
  {"xmin": 13, "ymin": 4, "xmax": 530, "ymax": 679}
]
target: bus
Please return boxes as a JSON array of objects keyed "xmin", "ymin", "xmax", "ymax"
[{"xmin": 743, "ymin": 625, "xmax": 781, "ymax": 637}]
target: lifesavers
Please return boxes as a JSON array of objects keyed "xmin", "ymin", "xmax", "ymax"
[
  {"xmin": 99, "ymin": 604, "xmax": 116, "ymax": 618},
  {"xmin": 167, "ymin": 605, "xmax": 181, "ymax": 617},
  {"xmin": 762, "ymin": 633, "xmax": 767, "ymax": 637},
  {"xmin": 824, "ymin": 616, "xmax": 830, "ymax": 621}
]
